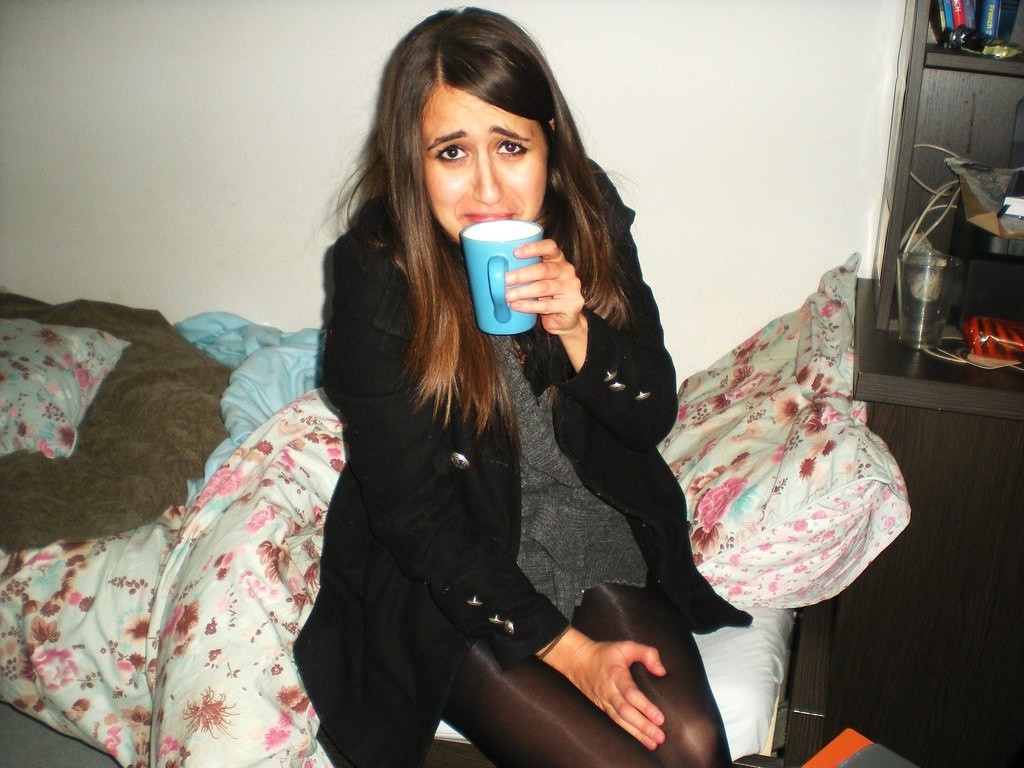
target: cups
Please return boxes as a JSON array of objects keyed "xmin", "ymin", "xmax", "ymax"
[
  {"xmin": 462, "ymin": 219, "xmax": 545, "ymax": 336},
  {"xmin": 898, "ymin": 250, "xmax": 961, "ymax": 350}
]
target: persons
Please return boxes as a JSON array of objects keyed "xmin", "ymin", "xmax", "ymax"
[{"xmin": 295, "ymin": 8, "xmax": 754, "ymax": 768}]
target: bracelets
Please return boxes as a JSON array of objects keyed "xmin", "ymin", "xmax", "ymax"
[{"xmin": 537, "ymin": 625, "xmax": 573, "ymax": 660}]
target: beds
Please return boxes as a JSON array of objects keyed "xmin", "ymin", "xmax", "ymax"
[{"xmin": 0, "ymin": 284, "xmax": 831, "ymax": 768}]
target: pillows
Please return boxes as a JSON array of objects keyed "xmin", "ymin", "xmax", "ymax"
[
  {"xmin": 655, "ymin": 252, "xmax": 912, "ymax": 612},
  {"xmin": 0, "ymin": 318, "xmax": 133, "ymax": 460}
]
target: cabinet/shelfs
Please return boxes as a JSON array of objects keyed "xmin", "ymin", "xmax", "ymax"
[{"xmin": 779, "ymin": 0, "xmax": 1024, "ymax": 768}]
